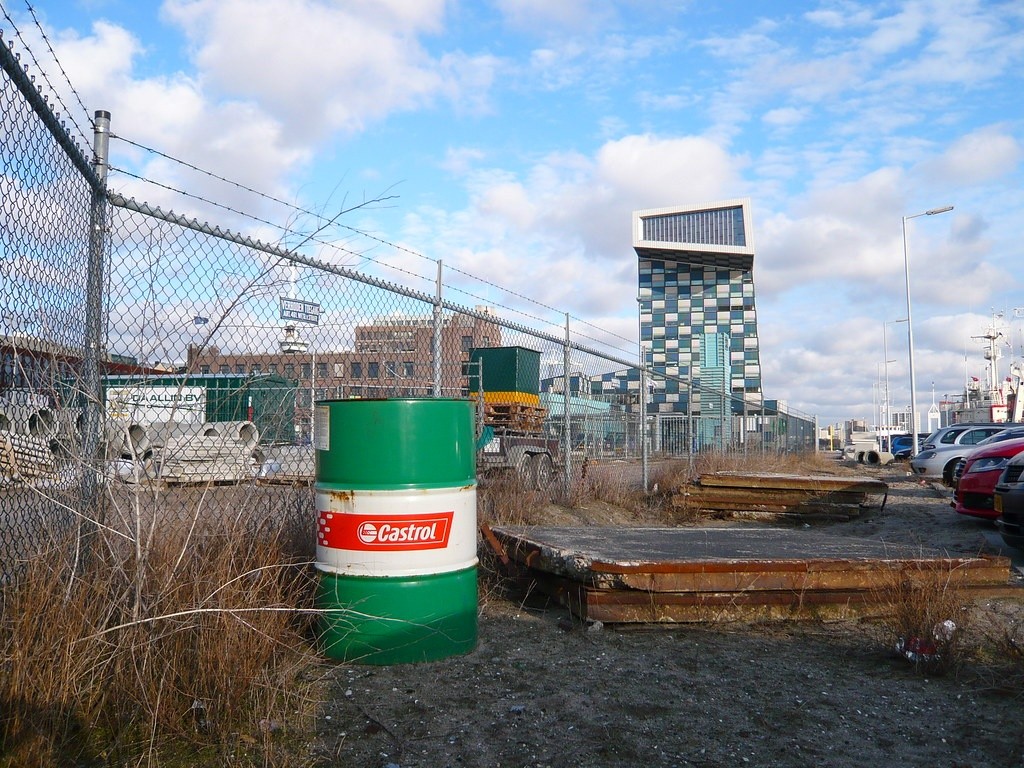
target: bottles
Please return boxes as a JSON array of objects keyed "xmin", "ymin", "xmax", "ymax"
[{"xmin": 896, "ymin": 618, "xmax": 956, "ymax": 663}]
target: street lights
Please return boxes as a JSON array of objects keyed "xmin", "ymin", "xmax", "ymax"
[
  {"xmin": 873, "ymin": 318, "xmax": 908, "ymax": 454},
  {"xmin": 901, "ymin": 205, "xmax": 954, "ymax": 460}
]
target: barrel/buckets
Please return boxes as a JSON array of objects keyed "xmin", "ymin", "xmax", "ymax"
[{"xmin": 311, "ymin": 397, "xmax": 482, "ymax": 665}]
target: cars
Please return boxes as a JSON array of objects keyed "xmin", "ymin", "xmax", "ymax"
[{"xmin": 882, "ymin": 422, "xmax": 1024, "ymax": 547}]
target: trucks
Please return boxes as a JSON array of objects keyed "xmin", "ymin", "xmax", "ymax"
[{"xmin": 474, "ymin": 427, "xmax": 565, "ymax": 490}]
